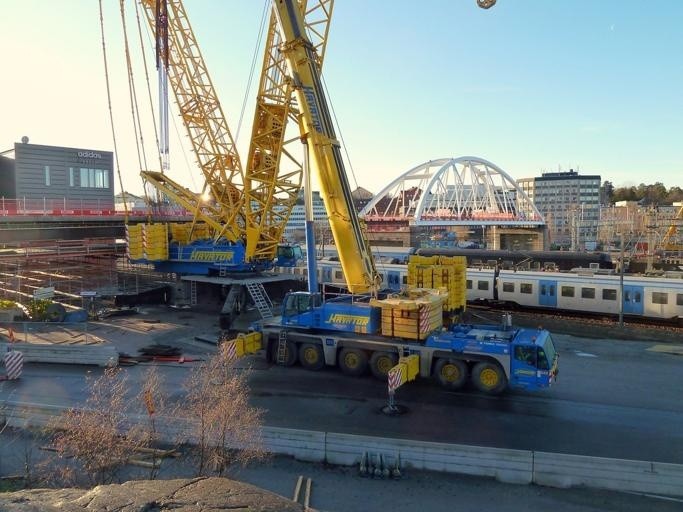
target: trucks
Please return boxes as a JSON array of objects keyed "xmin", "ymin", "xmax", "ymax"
[{"xmin": 260, "ymin": 314, "xmax": 559, "ymax": 395}]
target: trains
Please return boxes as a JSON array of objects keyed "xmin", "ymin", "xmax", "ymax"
[{"xmin": 270, "ymin": 251, "xmax": 681, "ymax": 327}]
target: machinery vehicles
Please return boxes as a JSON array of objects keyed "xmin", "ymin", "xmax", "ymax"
[{"xmin": 94, "ymin": 0, "xmax": 385, "ymax": 300}]
